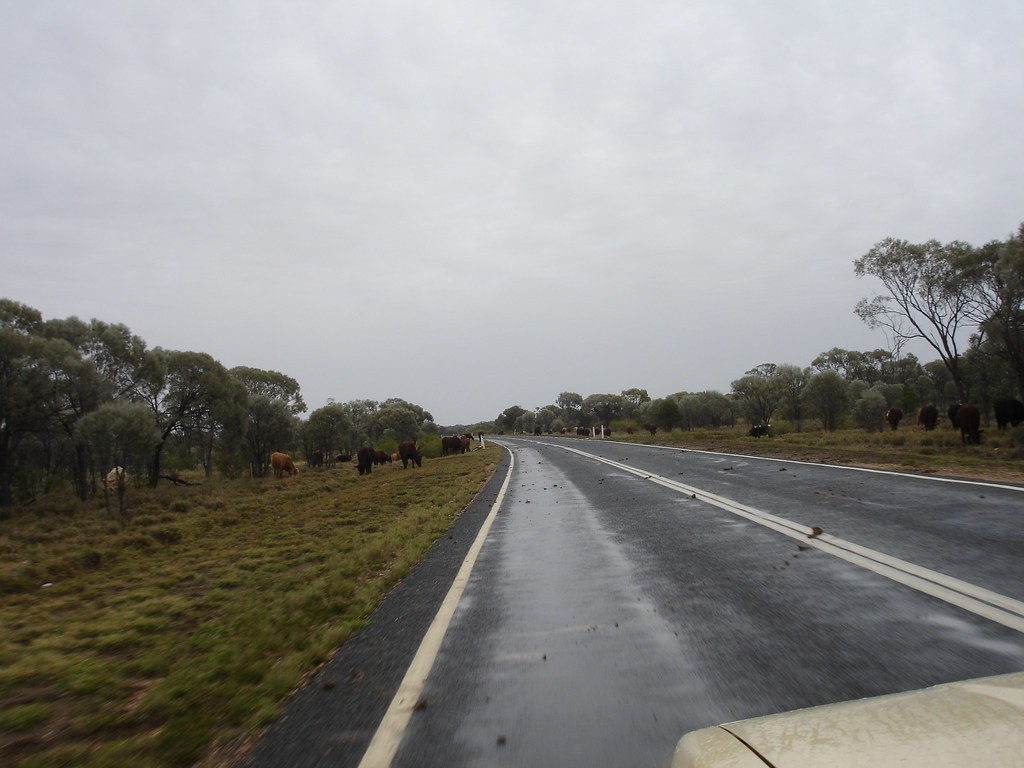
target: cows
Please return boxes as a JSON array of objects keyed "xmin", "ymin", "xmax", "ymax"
[
  {"xmin": 103, "ymin": 466, "xmax": 130, "ymax": 495},
  {"xmin": 442, "ymin": 433, "xmax": 474, "ymax": 454},
  {"xmin": 311, "ymin": 443, "xmax": 423, "ymax": 476},
  {"xmin": 271, "ymin": 453, "xmax": 298, "ymax": 478},
  {"xmin": 534, "ymin": 426, "xmax": 611, "ymax": 436},
  {"xmin": 886, "ymin": 400, "xmax": 1024, "ymax": 444},
  {"xmin": 478, "ymin": 431, "xmax": 485, "ymax": 442}
]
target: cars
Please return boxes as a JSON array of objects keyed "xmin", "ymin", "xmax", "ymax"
[{"xmin": 671, "ymin": 672, "xmax": 1024, "ymax": 767}]
